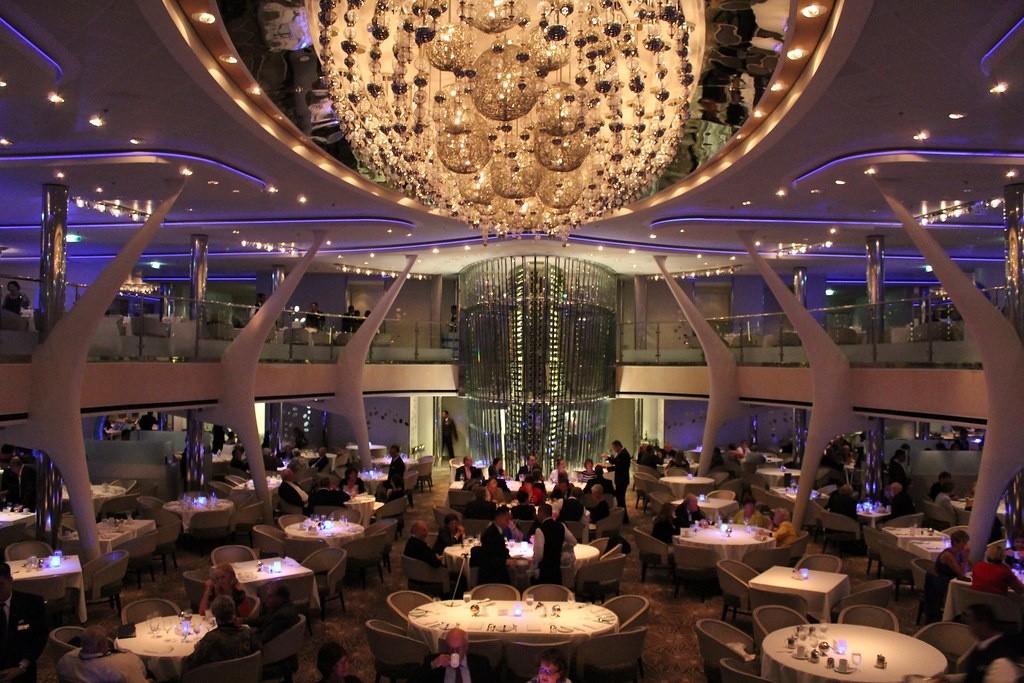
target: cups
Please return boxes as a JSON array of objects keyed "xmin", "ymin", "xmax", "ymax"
[
  {"xmin": 22, "ymin": 508, "xmax": 30, "ymax": 515},
  {"xmin": 839, "ymin": 659, "xmax": 850, "ymax": 673},
  {"xmin": 796, "ymin": 646, "xmax": 807, "ymax": 658},
  {"xmin": 449, "ymin": 653, "xmax": 460, "ymax": 668}
]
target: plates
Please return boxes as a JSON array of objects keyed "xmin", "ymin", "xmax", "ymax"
[
  {"xmin": 792, "ymin": 654, "xmax": 810, "ymax": 659},
  {"xmin": 835, "ymin": 666, "xmax": 854, "ymax": 673}
]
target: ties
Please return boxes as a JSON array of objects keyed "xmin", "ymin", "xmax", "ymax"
[
  {"xmin": 0, "ymin": 602, "xmax": 7, "ymax": 651},
  {"xmin": 455, "ymin": 667, "xmax": 463, "ymax": 683}
]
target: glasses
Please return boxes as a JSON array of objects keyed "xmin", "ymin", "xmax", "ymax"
[{"xmin": 540, "ymin": 668, "xmax": 560, "ymax": 676}]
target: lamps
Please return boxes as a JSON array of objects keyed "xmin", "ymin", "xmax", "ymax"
[{"xmin": 306, "ymin": 0, "xmax": 710, "ymax": 246}]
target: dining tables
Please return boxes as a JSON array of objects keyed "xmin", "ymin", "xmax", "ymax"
[
  {"xmin": 674, "ymin": 523, "xmax": 774, "ymax": 580},
  {"xmin": 343, "ymin": 493, "xmax": 376, "ymax": 526},
  {"xmin": 114, "ymin": 613, "xmax": 217, "ymax": 683},
  {"xmin": 661, "ymin": 461, "xmax": 700, "ymax": 478},
  {"xmin": 161, "ymin": 497, "xmax": 233, "ymax": 557},
  {"xmin": 359, "ymin": 473, "xmax": 390, "ymax": 494},
  {"xmin": 4, "ymin": 554, "xmax": 88, "ymax": 624},
  {"xmin": 0, "ymin": 513, "xmax": 38, "ymax": 547},
  {"xmin": 951, "ymin": 500, "xmax": 1006, "ymax": 525},
  {"xmin": 443, "ymin": 539, "xmax": 599, "ymax": 590},
  {"xmin": 406, "ymin": 599, "xmax": 619, "ymax": 665},
  {"xmin": 660, "ymin": 477, "xmax": 716, "ymax": 498},
  {"xmin": 760, "ymin": 456, "xmax": 784, "ymax": 468},
  {"xmin": 443, "ymin": 480, "xmax": 588, "ymax": 501},
  {"xmin": 282, "ymin": 522, "xmax": 365, "ymax": 548},
  {"xmin": 748, "ymin": 566, "xmax": 850, "ymax": 624},
  {"xmin": 299, "ymin": 451, "xmax": 338, "ymax": 474},
  {"xmin": 941, "ymin": 572, "xmax": 1024, "ymax": 622},
  {"xmin": 58, "ymin": 484, "xmax": 127, "ymax": 520},
  {"xmin": 758, "ymin": 623, "xmax": 947, "ymax": 683},
  {"xmin": 345, "ymin": 445, "xmax": 387, "ymax": 463},
  {"xmin": 757, "ymin": 468, "xmax": 801, "ymax": 487},
  {"xmin": 212, "ymin": 454, "xmax": 234, "ymax": 477},
  {"xmin": 907, "ymin": 539, "xmax": 952, "ymax": 561},
  {"xmin": 462, "ymin": 506, "xmax": 591, "ymax": 542},
  {"xmin": 232, "ymin": 477, "xmax": 281, "ymax": 506},
  {"xmin": 784, "ymin": 492, "xmax": 831, "ymax": 510},
  {"xmin": 61, "ymin": 517, "xmax": 155, "ymax": 561},
  {"xmin": 231, "ymin": 556, "xmax": 321, "ymax": 636},
  {"xmin": 370, "ymin": 457, "xmax": 417, "ymax": 474},
  {"xmin": 768, "ymin": 485, "xmax": 790, "ymax": 497},
  {"xmin": 880, "ymin": 526, "xmax": 949, "ymax": 548},
  {"xmin": 857, "ymin": 509, "xmax": 892, "ymax": 530}
]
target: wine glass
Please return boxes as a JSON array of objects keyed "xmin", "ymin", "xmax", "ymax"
[
  {"xmin": 689, "ymin": 515, "xmax": 755, "ymax": 536},
  {"xmin": 433, "ymin": 597, "xmax": 440, "ymax": 616},
  {"xmin": 463, "ymin": 591, "xmax": 471, "ymax": 608},
  {"xmin": 149, "ymin": 618, "xmax": 203, "ymax": 643},
  {"xmin": 526, "ymin": 593, "xmax": 534, "ymax": 613},
  {"xmin": 800, "ymin": 620, "xmax": 861, "ymax": 672}
]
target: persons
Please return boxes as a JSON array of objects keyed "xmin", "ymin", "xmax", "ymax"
[
  {"xmin": 527, "ymin": 647, "xmax": 571, "ymax": 683},
  {"xmin": 421, "ymin": 627, "xmax": 491, "ymax": 683},
  {"xmin": 342, "ymin": 306, "xmax": 371, "ymax": 333},
  {"xmin": 185, "ymin": 563, "xmax": 298, "ymax": 683},
  {"xmin": 450, "ymin": 305, "xmax": 456, "ymax": 332},
  {"xmin": 212, "ymin": 424, "xmax": 247, "ymax": 472},
  {"xmin": 262, "ymin": 427, "xmax": 406, "ymax": 515},
  {"xmin": 637, "ymin": 439, "xmax": 799, "ymax": 548},
  {"xmin": 249, "ymin": 293, "xmax": 266, "ymax": 318},
  {"xmin": 404, "ymin": 439, "xmax": 630, "ymax": 596},
  {"xmin": 315, "ymin": 642, "xmax": 362, "ymax": 683},
  {"xmin": 57, "ymin": 626, "xmax": 126, "ymax": 683},
  {"xmin": 2, "ymin": 280, "xmax": 30, "ymax": 316},
  {"xmin": 820, "ymin": 429, "xmax": 1024, "ymax": 616},
  {"xmin": 0, "ymin": 562, "xmax": 49, "ymax": 683},
  {"xmin": 307, "ymin": 302, "xmax": 325, "ymax": 329},
  {"xmin": 441, "ymin": 411, "xmax": 459, "ymax": 459},
  {"xmin": 931, "ymin": 603, "xmax": 1018, "ymax": 683},
  {"xmin": 104, "ymin": 411, "xmax": 158, "ymax": 442},
  {"xmin": 0, "ymin": 457, "xmax": 39, "ymax": 510}
]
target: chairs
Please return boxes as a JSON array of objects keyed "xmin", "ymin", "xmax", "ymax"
[{"xmin": 0, "ymin": 306, "xmax": 1024, "ymax": 683}]
